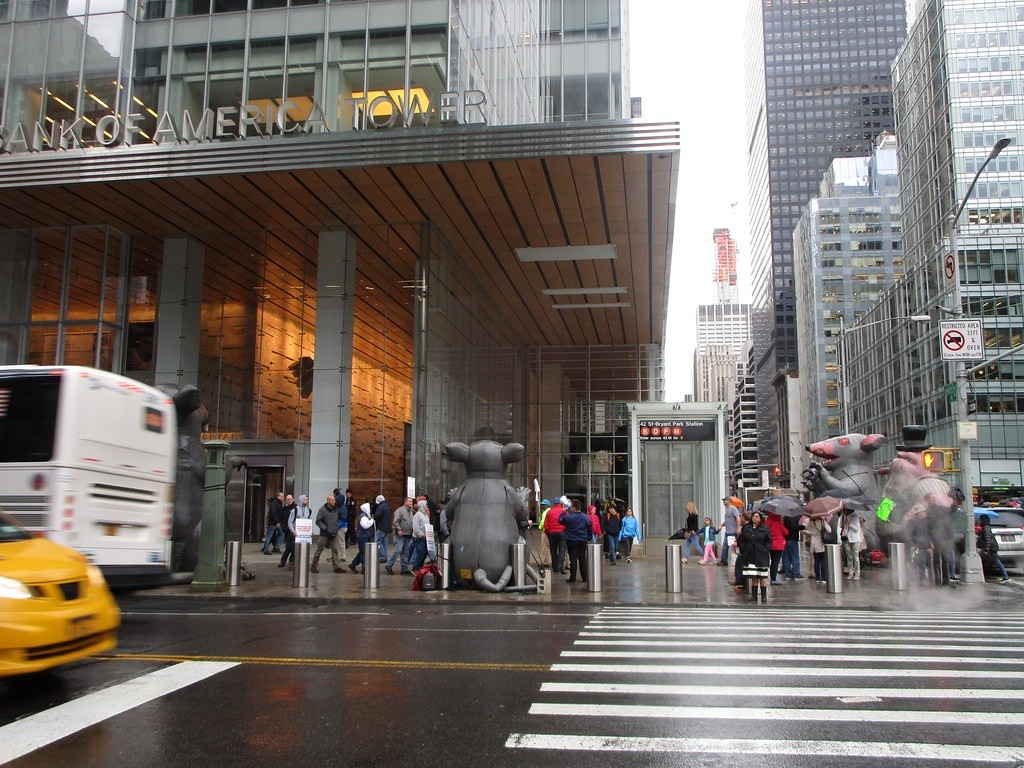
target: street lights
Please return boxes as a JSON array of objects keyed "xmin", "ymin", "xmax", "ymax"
[
  {"xmin": 944, "ymin": 137, "xmax": 1011, "ymax": 583},
  {"xmin": 838, "ymin": 315, "xmax": 932, "ymax": 437}
]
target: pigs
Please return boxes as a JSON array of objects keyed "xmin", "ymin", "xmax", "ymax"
[{"xmin": 875, "ymin": 442, "xmax": 954, "ymax": 559}]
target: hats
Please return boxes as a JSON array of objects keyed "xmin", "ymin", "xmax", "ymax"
[
  {"xmin": 722, "ymin": 496, "xmax": 732, "ymax": 502},
  {"xmin": 539, "ymin": 500, "xmax": 551, "ymax": 507},
  {"xmin": 914, "ymin": 504, "xmax": 926, "ymax": 514},
  {"xmin": 554, "ymin": 498, "xmax": 560, "ymax": 503}
]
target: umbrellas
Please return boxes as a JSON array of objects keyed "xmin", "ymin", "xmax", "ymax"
[
  {"xmin": 804, "ymin": 496, "xmax": 844, "ymax": 518},
  {"xmin": 820, "ymin": 488, "xmax": 878, "ymax": 511},
  {"xmin": 948, "ymin": 487, "xmax": 965, "ymax": 500},
  {"xmin": 798, "ymin": 514, "xmax": 833, "ymax": 526},
  {"xmin": 974, "ymin": 508, "xmax": 1000, "ymax": 520},
  {"xmin": 761, "ymin": 495, "xmax": 804, "ymax": 517},
  {"xmin": 730, "ymin": 497, "xmax": 744, "ymax": 507},
  {"xmin": 666, "ymin": 527, "xmax": 686, "ymax": 542}
]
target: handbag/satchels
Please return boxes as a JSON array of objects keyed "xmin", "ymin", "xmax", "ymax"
[{"xmin": 821, "ymin": 521, "xmax": 833, "ymax": 543}]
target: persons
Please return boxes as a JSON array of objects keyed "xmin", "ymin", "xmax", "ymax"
[
  {"xmin": 741, "ymin": 512, "xmax": 772, "ymax": 602},
  {"xmin": 904, "ymin": 492, "xmax": 1009, "ymax": 587},
  {"xmin": 681, "ymin": 501, "xmax": 704, "ymax": 563},
  {"xmin": 263, "ymin": 488, "xmax": 446, "ymax": 575},
  {"xmin": 538, "ymin": 496, "xmax": 641, "ymax": 582},
  {"xmin": 719, "ymin": 493, "xmax": 866, "ymax": 591},
  {"xmin": 693, "ymin": 517, "xmax": 720, "ymax": 565}
]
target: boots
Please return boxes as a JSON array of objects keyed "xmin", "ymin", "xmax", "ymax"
[
  {"xmin": 749, "ymin": 586, "xmax": 758, "ymax": 601},
  {"xmin": 760, "ymin": 586, "xmax": 768, "ymax": 602}
]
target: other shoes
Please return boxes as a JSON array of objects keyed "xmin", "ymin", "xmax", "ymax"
[
  {"xmin": 933, "ymin": 574, "xmax": 1010, "ymax": 588},
  {"xmin": 604, "ymin": 554, "xmax": 609, "ymax": 558},
  {"xmin": 710, "ymin": 560, "xmax": 718, "ymax": 565},
  {"xmin": 626, "ymin": 557, "xmax": 632, "ymax": 563},
  {"xmin": 738, "ymin": 586, "xmax": 747, "ymax": 590},
  {"xmin": 681, "ymin": 559, "xmax": 688, "ymax": 563},
  {"xmin": 770, "ymin": 570, "xmax": 861, "ymax": 585},
  {"xmin": 566, "ymin": 579, "xmax": 575, "ymax": 583},
  {"xmin": 698, "ymin": 560, "xmax": 706, "ymax": 564},
  {"xmin": 717, "ymin": 561, "xmax": 728, "ymax": 566},
  {"xmin": 612, "ymin": 561, "xmax": 617, "ymax": 565},
  {"xmin": 561, "ymin": 570, "xmax": 568, "ymax": 574},
  {"xmin": 616, "ymin": 554, "xmax": 621, "ymax": 559},
  {"xmin": 261, "ymin": 543, "xmax": 422, "ymax": 575}
]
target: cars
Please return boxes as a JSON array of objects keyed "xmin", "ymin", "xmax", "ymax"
[
  {"xmin": 972, "ymin": 488, "xmax": 1024, "ymax": 577},
  {"xmin": 0, "ymin": 510, "xmax": 122, "ymax": 676}
]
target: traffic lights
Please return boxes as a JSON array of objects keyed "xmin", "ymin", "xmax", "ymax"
[{"xmin": 921, "ymin": 450, "xmax": 945, "ymax": 471}]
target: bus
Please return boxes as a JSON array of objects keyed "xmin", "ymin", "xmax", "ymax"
[{"xmin": 0, "ymin": 364, "xmax": 179, "ymax": 598}]
